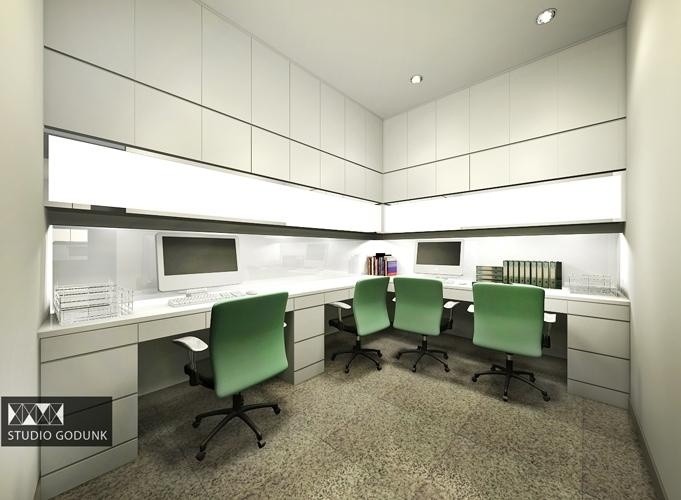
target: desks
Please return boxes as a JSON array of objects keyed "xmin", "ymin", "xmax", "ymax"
[{"xmin": 39, "ymin": 271, "xmax": 630, "ymax": 499}]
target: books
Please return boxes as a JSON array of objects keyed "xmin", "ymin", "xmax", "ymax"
[{"xmin": 368, "ymin": 253, "xmax": 398, "ymax": 276}]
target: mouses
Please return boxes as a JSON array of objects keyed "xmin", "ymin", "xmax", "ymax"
[{"xmin": 246, "ymin": 290, "xmax": 257, "ymax": 295}]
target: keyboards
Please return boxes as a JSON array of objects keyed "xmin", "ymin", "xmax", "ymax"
[{"xmin": 168, "ymin": 290, "xmax": 242, "ymax": 307}]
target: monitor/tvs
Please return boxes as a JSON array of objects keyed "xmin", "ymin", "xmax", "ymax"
[
  {"xmin": 155, "ymin": 232, "xmax": 241, "ymax": 295},
  {"xmin": 413, "ymin": 239, "xmax": 464, "ymax": 276}
]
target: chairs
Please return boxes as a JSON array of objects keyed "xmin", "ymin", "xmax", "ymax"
[{"xmin": 171, "ymin": 275, "xmax": 557, "ymax": 460}]
